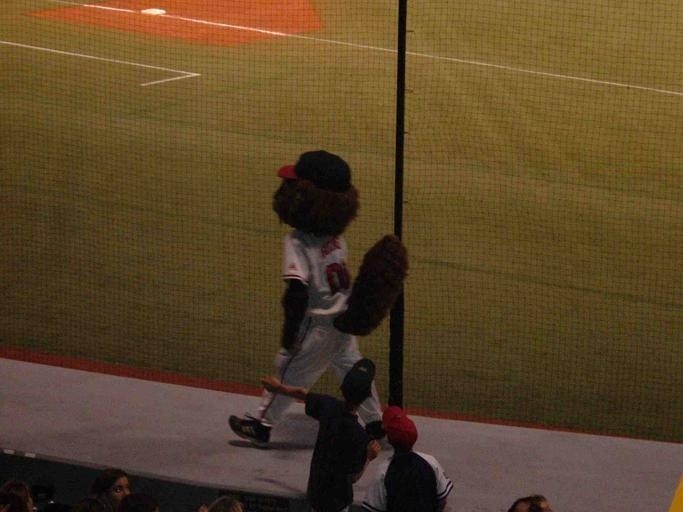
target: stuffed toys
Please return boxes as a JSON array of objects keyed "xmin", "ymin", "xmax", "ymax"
[{"xmin": 227, "ymin": 149, "xmax": 410, "ymax": 449}]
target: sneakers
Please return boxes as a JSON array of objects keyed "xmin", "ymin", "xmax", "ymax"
[
  {"xmin": 367, "ymin": 421, "xmax": 386, "ymax": 440},
  {"xmin": 229, "ymin": 415, "xmax": 271, "ymax": 448}
]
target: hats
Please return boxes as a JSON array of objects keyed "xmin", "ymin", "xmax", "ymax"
[
  {"xmin": 383, "ymin": 408, "xmax": 417, "ymax": 449},
  {"xmin": 278, "ymin": 151, "xmax": 351, "ymax": 192},
  {"xmin": 339, "ymin": 359, "xmax": 376, "ymax": 403}
]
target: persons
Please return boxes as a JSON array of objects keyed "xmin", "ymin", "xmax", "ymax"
[
  {"xmin": 503, "ymin": 493, "xmax": 557, "ymax": 512},
  {"xmin": 259, "ymin": 357, "xmax": 382, "ymax": 512},
  {"xmin": 0, "ymin": 466, "xmax": 248, "ymax": 512},
  {"xmin": 356, "ymin": 404, "xmax": 455, "ymax": 512}
]
